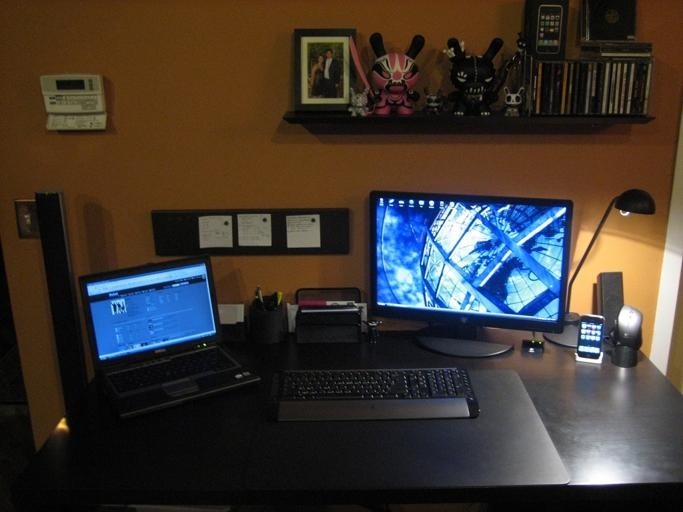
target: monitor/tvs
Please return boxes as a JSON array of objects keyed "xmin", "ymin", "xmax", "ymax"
[{"xmin": 369, "ymin": 190, "xmax": 573, "ymax": 358}]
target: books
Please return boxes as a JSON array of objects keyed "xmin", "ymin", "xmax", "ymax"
[{"xmin": 527, "ymin": 39, "xmax": 652, "ymax": 118}]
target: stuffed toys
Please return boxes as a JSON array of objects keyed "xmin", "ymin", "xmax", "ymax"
[{"xmin": 347, "ymin": 29, "xmax": 530, "ymax": 117}]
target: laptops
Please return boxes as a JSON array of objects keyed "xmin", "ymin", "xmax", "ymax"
[{"xmin": 78, "ymin": 255, "xmax": 263, "ymax": 420}]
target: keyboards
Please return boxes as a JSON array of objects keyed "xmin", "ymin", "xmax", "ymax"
[{"xmin": 266, "ymin": 366, "xmax": 479, "ymax": 422}]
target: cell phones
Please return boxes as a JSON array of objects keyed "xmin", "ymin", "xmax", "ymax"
[
  {"xmin": 535, "ymin": 4, "xmax": 563, "ymax": 55},
  {"xmin": 577, "ymin": 314, "xmax": 605, "ymax": 359}
]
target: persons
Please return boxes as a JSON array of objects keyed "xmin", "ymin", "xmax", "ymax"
[
  {"xmin": 321, "ymin": 49, "xmax": 343, "ymax": 99},
  {"xmin": 310, "ymin": 52, "xmax": 327, "ymax": 99}
]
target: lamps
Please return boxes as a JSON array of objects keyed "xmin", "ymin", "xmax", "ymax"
[{"xmin": 543, "ymin": 189, "xmax": 654, "ymax": 348}]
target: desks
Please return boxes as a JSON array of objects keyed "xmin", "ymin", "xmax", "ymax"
[{"xmin": 7, "ymin": 327, "xmax": 682, "ymax": 512}]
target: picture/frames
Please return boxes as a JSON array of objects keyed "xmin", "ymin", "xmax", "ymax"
[{"xmin": 294, "ymin": 29, "xmax": 356, "ymax": 111}]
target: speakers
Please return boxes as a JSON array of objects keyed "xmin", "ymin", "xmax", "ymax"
[
  {"xmin": 596, "ymin": 272, "xmax": 623, "ymax": 351},
  {"xmin": 35, "ymin": 190, "xmax": 88, "ymax": 413}
]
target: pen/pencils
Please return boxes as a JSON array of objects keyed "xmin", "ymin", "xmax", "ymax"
[
  {"xmin": 255, "ymin": 287, "xmax": 283, "ymax": 308},
  {"xmin": 299, "ymin": 299, "xmax": 354, "ymax": 305}
]
